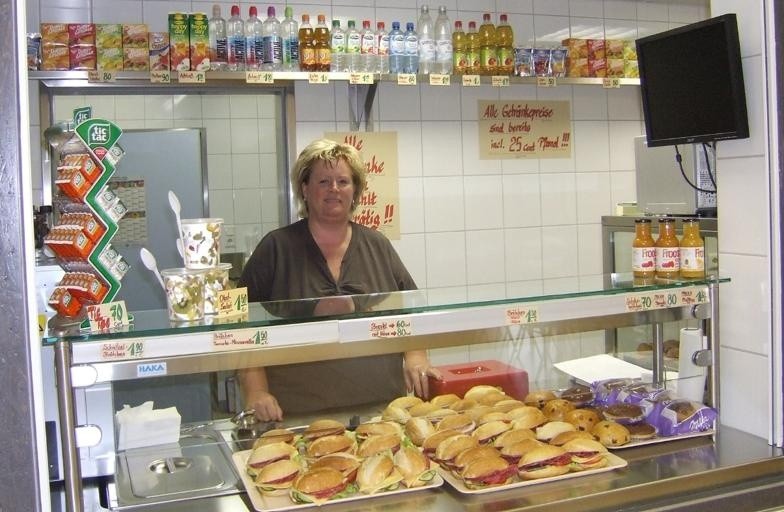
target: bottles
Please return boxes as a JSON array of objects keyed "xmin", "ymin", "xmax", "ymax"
[
  {"xmin": 282, "ymin": 7, "xmax": 299, "ymax": 72},
  {"xmin": 329, "ymin": 20, "xmax": 346, "ymax": 72},
  {"xmin": 376, "ymin": 21, "xmax": 389, "ymax": 73},
  {"xmin": 631, "ymin": 216, "xmax": 656, "ymax": 280},
  {"xmin": 346, "ymin": 20, "xmax": 360, "ymax": 73},
  {"xmin": 314, "ymin": 14, "xmax": 330, "ymax": 72},
  {"xmin": 435, "ymin": 5, "xmax": 453, "ymax": 75},
  {"xmin": 466, "ymin": 21, "xmax": 481, "ymax": 75},
  {"xmin": 360, "ymin": 21, "xmax": 376, "ymax": 73},
  {"xmin": 298, "ymin": 14, "xmax": 315, "ymax": 72},
  {"xmin": 244, "ymin": 6, "xmax": 263, "ymax": 71},
  {"xmin": 678, "ymin": 217, "xmax": 707, "ymax": 280},
  {"xmin": 227, "ymin": 6, "xmax": 245, "ymax": 73},
  {"xmin": 480, "ymin": 14, "xmax": 498, "ymax": 75},
  {"xmin": 496, "ymin": 14, "xmax": 514, "ymax": 76},
  {"xmin": 263, "ymin": 6, "xmax": 283, "ymax": 72},
  {"xmin": 389, "ymin": 21, "xmax": 404, "ymax": 73},
  {"xmin": 417, "ymin": 4, "xmax": 436, "ymax": 74},
  {"xmin": 208, "ymin": 4, "xmax": 227, "ymax": 72},
  {"xmin": 654, "ymin": 215, "xmax": 680, "ymax": 280},
  {"xmin": 452, "ymin": 20, "xmax": 466, "ymax": 75},
  {"xmin": 404, "ymin": 23, "xmax": 418, "ymax": 74}
]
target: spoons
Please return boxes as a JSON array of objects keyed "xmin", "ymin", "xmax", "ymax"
[
  {"xmin": 167, "ymin": 190, "xmax": 187, "ymax": 264},
  {"xmin": 174, "ymin": 237, "xmax": 183, "ymax": 258},
  {"xmin": 139, "ymin": 247, "xmax": 165, "ymax": 294}
]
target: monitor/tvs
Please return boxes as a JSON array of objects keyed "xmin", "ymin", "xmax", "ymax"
[{"xmin": 634, "ymin": 13, "xmax": 749, "ymax": 148}]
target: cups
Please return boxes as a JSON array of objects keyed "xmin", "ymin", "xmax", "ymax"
[
  {"xmin": 203, "ymin": 265, "xmax": 237, "ymax": 314},
  {"xmin": 162, "ymin": 269, "xmax": 209, "ymax": 321},
  {"xmin": 182, "ymin": 217, "xmax": 226, "ymax": 270}
]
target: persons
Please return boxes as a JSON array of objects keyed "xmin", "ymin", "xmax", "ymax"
[{"xmin": 237, "ymin": 139, "xmax": 444, "ymax": 422}]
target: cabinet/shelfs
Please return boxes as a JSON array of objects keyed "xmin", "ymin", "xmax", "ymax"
[
  {"xmin": 44, "ymin": 115, "xmax": 140, "ymax": 330},
  {"xmin": 3, "ymin": 0, "xmax": 783, "ymax": 512}
]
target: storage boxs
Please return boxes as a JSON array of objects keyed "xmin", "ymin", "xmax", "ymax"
[{"xmin": 415, "ymin": 359, "xmax": 531, "ymax": 404}]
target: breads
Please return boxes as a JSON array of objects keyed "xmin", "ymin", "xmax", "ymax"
[{"xmin": 525, "ymin": 378, "xmax": 719, "ymax": 446}]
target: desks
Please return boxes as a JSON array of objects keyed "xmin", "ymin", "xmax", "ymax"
[{"xmin": 548, "ymin": 354, "xmax": 655, "ymax": 389}]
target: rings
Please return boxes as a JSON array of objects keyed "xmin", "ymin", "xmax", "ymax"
[{"xmin": 419, "ymin": 371, "xmax": 427, "ymax": 376}]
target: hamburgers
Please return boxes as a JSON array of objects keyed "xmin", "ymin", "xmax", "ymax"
[{"xmin": 246, "ymin": 384, "xmax": 609, "ymax": 505}]
